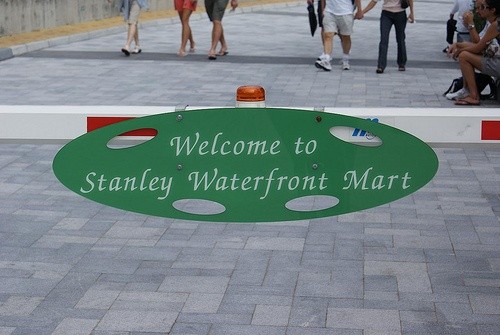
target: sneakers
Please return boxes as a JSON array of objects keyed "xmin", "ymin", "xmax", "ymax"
[
  {"xmin": 316, "ymin": 60, "xmax": 332, "ymax": 70},
  {"xmin": 343, "ymin": 61, "xmax": 350, "ymax": 69},
  {"xmin": 446, "ymin": 88, "xmax": 470, "ymax": 100}
]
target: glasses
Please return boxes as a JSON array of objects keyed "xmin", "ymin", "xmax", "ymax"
[{"xmin": 480, "ymin": 4, "xmax": 495, "ymax": 10}]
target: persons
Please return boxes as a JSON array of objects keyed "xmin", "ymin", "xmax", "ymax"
[
  {"xmin": 307, "ymin": 0, "xmax": 364, "ymax": 71},
  {"xmin": 354, "ymin": 0, "xmax": 414, "ymax": 73},
  {"xmin": 204, "ymin": 0, "xmax": 238, "ymax": 59},
  {"xmin": 107, "ymin": 0, "xmax": 148, "ymax": 55},
  {"xmin": 174, "ymin": 0, "xmax": 198, "ymax": 58},
  {"xmin": 443, "ymin": 0, "xmax": 500, "ymax": 105}
]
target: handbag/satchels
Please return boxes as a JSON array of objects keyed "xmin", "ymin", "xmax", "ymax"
[
  {"xmin": 307, "ymin": 4, "xmax": 317, "ymax": 36},
  {"xmin": 446, "ymin": 16, "xmax": 456, "ymax": 44},
  {"xmin": 444, "ymin": 72, "xmax": 493, "ymax": 99}
]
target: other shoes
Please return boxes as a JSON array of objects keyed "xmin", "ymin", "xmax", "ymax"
[
  {"xmin": 376, "ymin": 68, "xmax": 383, "ymax": 73},
  {"xmin": 178, "ymin": 50, "xmax": 186, "ymax": 56},
  {"xmin": 189, "ymin": 43, "xmax": 195, "ymax": 52},
  {"xmin": 318, "ymin": 52, "xmax": 324, "ymax": 60},
  {"xmin": 132, "ymin": 47, "xmax": 141, "ymax": 53},
  {"xmin": 122, "ymin": 48, "xmax": 130, "ymax": 55},
  {"xmin": 399, "ymin": 66, "xmax": 405, "ymax": 71},
  {"xmin": 443, "ymin": 47, "xmax": 452, "ymax": 53}
]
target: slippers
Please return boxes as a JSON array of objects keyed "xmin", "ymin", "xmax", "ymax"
[
  {"xmin": 454, "ymin": 98, "xmax": 480, "ymax": 105},
  {"xmin": 208, "ymin": 54, "xmax": 216, "ymax": 58},
  {"xmin": 216, "ymin": 51, "xmax": 229, "ymax": 56}
]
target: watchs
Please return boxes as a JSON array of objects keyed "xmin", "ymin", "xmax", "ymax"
[{"xmin": 467, "ymin": 25, "xmax": 475, "ymax": 30}]
